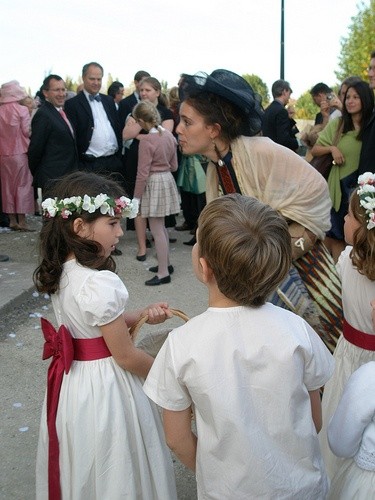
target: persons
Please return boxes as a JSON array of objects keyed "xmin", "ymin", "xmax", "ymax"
[
  {"xmin": 118, "ymin": 71, "xmax": 210, "ymax": 286},
  {"xmin": 175, "ymin": 69, "xmax": 343, "ymax": 402},
  {"xmin": 260, "ymin": 48, "xmax": 375, "ymax": 264},
  {"xmin": 0, "ymin": 62, "xmax": 122, "ymax": 231},
  {"xmin": 32, "ymin": 171, "xmax": 337, "ymax": 500},
  {"xmin": 317, "ymin": 172, "xmax": 375, "ymax": 500}
]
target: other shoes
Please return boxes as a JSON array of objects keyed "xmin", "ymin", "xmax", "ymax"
[
  {"xmin": 146, "ymin": 239, "xmax": 151, "ymax": 248},
  {"xmin": 111, "ymin": 247, "xmax": 121, "ymax": 256},
  {"xmin": 0, "ymin": 255, "xmax": 9, "ymax": 262},
  {"xmin": 175, "ymin": 223, "xmax": 190, "ymax": 231},
  {"xmin": 169, "ymin": 238, "xmax": 176, "ymax": 243},
  {"xmin": 148, "ymin": 265, "xmax": 174, "ymax": 275},
  {"xmin": 145, "ymin": 274, "xmax": 170, "ymax": 286},
  {"xmin": 183, "ymin": 236, "xmax": 196, "ymax": 246},
  {"xmin": 137, "ymin": 255, "xmax": 146, "ymax": 261}
]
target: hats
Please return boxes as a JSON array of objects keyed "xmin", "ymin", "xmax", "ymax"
[
  {"xmin": 178, "ymin": 68, "xmax": 265, "ymax": 137},
  {"xmin": 0, "ymin": 80, "xmax": 26, "ymax": 102}
]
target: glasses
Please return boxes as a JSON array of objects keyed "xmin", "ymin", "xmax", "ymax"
[
  {"xmin": 47, "ymin": 88, "xmax": 67, "ymax": 93},
  {"xmin": 367, "ymin": 65, "xmax": 375, "ymax": 71}
]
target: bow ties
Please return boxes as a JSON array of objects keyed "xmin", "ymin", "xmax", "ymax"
[{"xmin": 89, "ymin": 95, "xmax": 101, "ymax": 102}]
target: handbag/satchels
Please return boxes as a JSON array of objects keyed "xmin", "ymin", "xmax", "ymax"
[{"xmin": 309, "ymin": 117, "xmax": 344, "ymax": 184}]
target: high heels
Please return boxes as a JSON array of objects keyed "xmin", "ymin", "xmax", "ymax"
[
  {"xmin": 18, "ymin": 223, "xmax": 36, "ymax": 231},
  {"xmin": 10, "ymin": 223, "xmax": 19, "ymax": 231}
]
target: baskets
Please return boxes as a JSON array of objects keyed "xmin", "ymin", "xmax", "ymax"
[{"xmin": 129, "ymin": 309, "xmax": 190, "ymax": 358}]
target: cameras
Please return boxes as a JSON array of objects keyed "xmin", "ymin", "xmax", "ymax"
[{"xmin": 326, "ymin": 95, "xmax": 334, "ymax": 100}]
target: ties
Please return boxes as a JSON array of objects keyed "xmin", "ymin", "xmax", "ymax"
[{"xmin": 58, "ymin": 109, "xmax": 74, "ymax": 139}]
